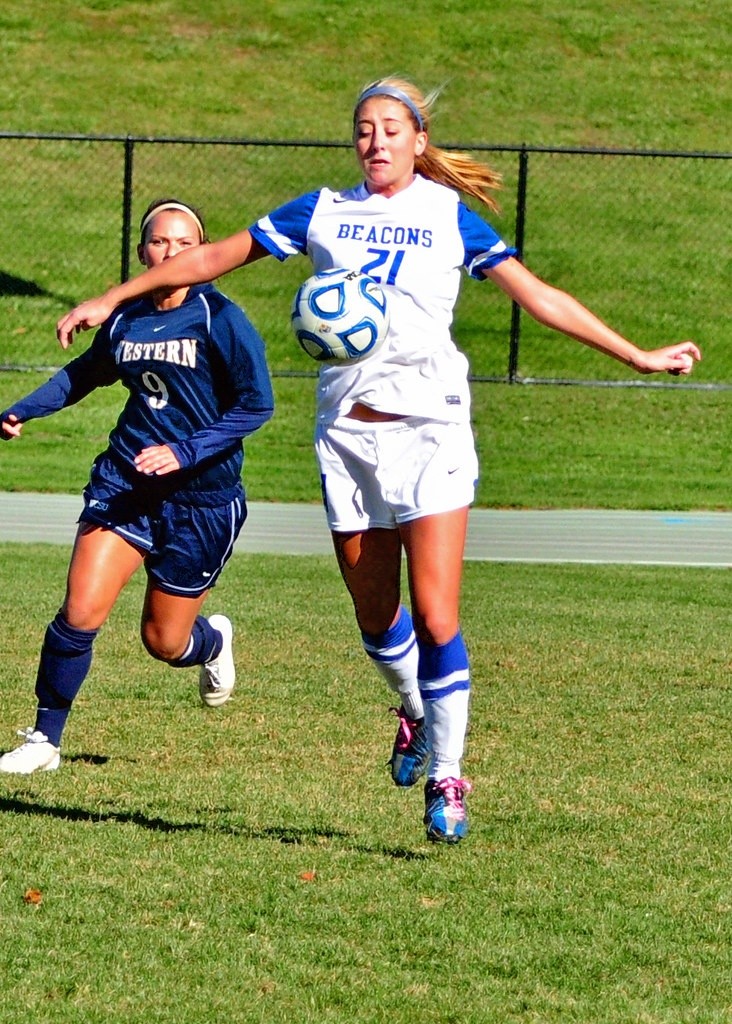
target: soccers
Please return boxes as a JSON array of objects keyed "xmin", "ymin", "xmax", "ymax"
[{"xmin": 291, "ymin": 266, "xmax": 390, "ymax": 364}]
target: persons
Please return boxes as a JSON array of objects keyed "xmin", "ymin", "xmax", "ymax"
[
  {"xmin": 55, "ymin": 77, "xmax": 701, "ymax": 843},
  {"xmin": 0, "ymin": 199, "xmax": 274, "ymax": 774}
]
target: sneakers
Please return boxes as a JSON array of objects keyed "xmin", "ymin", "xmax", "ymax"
[
  {"xmin": 0, "ymin": 727, "xmax": 61, "ymax": 773},
  {"xmin": 199, "ymin": 614, "xmax": 235, "ymax": 706},
  {"xmin": 388, "ymin": 703, "xmax": 431, "ymax": 787},
  {"xmin": 424, "ymin": 776, "xmax": 472, "ymax": 845}
]
